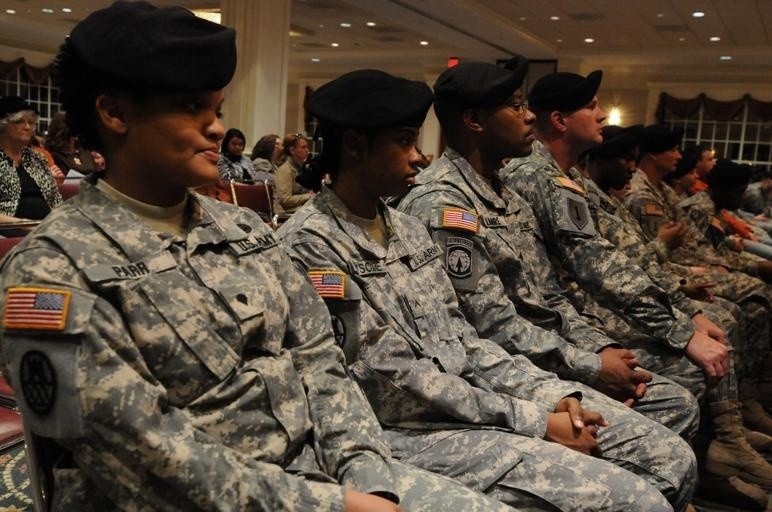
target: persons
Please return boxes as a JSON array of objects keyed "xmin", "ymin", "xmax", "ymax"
[
  {"xmin": 0, "ymin": 94, "xmax": 104, "ymax": 229},
  {"xmin": 398, "ymin": 58, "xmax": 699, "ymax": 442},
  {"xmin": 0, "ymin": 0, "xmax": 513, "ymax": 512},
  {"xmin": 214, "ymin": 129, "xmax": 316, "ymax": 221},
  {"xmin": 500, "ymin": 70, "xmax": 772, "ymax": 495},
  {"xmin": 277, "ymin": 69, "xmax": 698, "ymax": 512}
]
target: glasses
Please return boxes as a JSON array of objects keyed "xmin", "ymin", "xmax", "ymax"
[{"xmin": 503, "ymin": 98, "xmax": 531, "ymax": 113}]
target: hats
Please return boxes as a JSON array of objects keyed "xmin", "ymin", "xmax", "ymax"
[
  {"xmin": 305, "ymin": 67, "xmax": 436, "ymax": 132},
  {"xmin": 46, "ymin": 0, "xmax": 240, "ymax": 96},
  {"xmin": 433, "ymin": 54, "xmax": 530, "ymax": 121},
  {"xmin": 641, "ymin": 126, "xmax": 685, "ymax": 153},
  {"xmin": 0, "ymin": 95, "xmax": 39, "ymax": 119},
  {"xmin": 590, "ymin": 124, "xmax": 650, "ymax": 158},
  {"xmin": 528, "ymin": 69, "xmax": 604, "ymax": 113}
]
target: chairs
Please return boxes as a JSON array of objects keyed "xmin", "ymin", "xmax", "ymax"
[
  {"xmin": 0, "ymin": 354, "xmax": 28, "ymax": 457},
  {"xmin": 1, "ymin": 170, "xmax": 87, "ymax": 263},
  {"xmin": 198, "ymin": 177, "xmax": 292, "ymax": 230}
]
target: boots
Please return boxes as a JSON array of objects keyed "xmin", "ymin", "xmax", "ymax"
[{"xmin": 694, "ymin": 371, "xmax": 772, "ymax": 511}]
